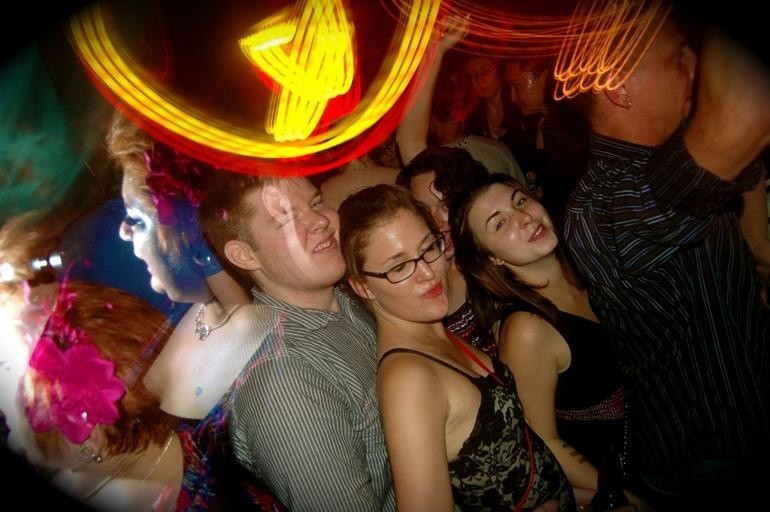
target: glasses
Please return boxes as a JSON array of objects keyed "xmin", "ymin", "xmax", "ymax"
[{"xmin": 358, "ymin": 230, "xmax": 447, "ymax": 284}]
